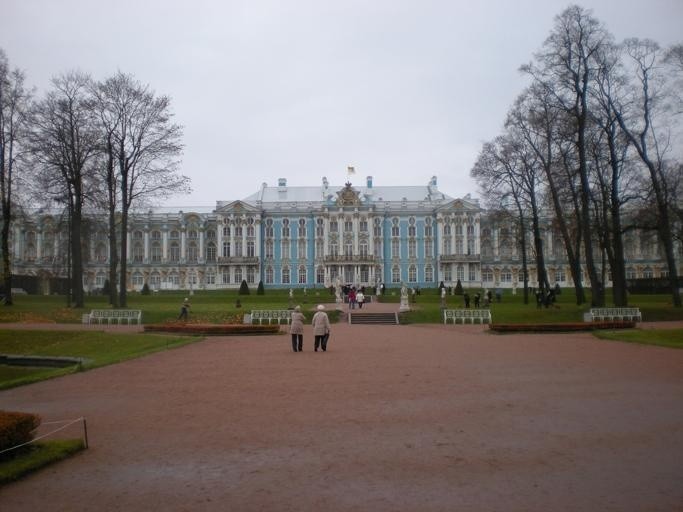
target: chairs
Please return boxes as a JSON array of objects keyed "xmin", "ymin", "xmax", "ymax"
[
  {"xmin": 588, "ymin": 307, "xmax": 642, "ymax": 324},
  {"xmin": 442, "ymin": 309, "xmax": 492, "ymax": 325},
  {"xmin": 88, "ymin": 308, "xmax": 142, "ymax": 328},
  {"xmin": 250, "ymin": 309, "xmax": 294, "ymax": 326}
]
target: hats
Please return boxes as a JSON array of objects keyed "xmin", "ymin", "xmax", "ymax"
[{"xmin": 317, "ymin": 304, "xmax": 325, "ymax": 310}]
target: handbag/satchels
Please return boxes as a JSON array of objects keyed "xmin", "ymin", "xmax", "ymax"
[{"xmin": 321, "ymin": 329, "xmax": 330, "ymax": 345}]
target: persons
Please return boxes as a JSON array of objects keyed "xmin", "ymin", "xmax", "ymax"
[
  {"xmin": 383, "ymin": 284, "xmax": 385, "ymax": 295},
  {"xmin": 344, "ymin": 285, "xmax": 364, "ymax": 309},
  {"xmin": 177, "ymin": 297, "xmax": 191, "ymax": 321},
  {"xmin": 535, "ymin": 288, "xmax": 556, "ymax": 312},
  {"xmin": 417, "ymin": 286, "xmax": 421, "ymax": 296},
  {"xmin": 464, "ymin": 289, "xmax": 501, "ymax": 310},
  {"xmin": 290, "ymin": 305, "xmax": 306, "ymax": 352},
  {"xmin": 312, "ymin": 305, "xmax": 331, "ymax": 351}
]
